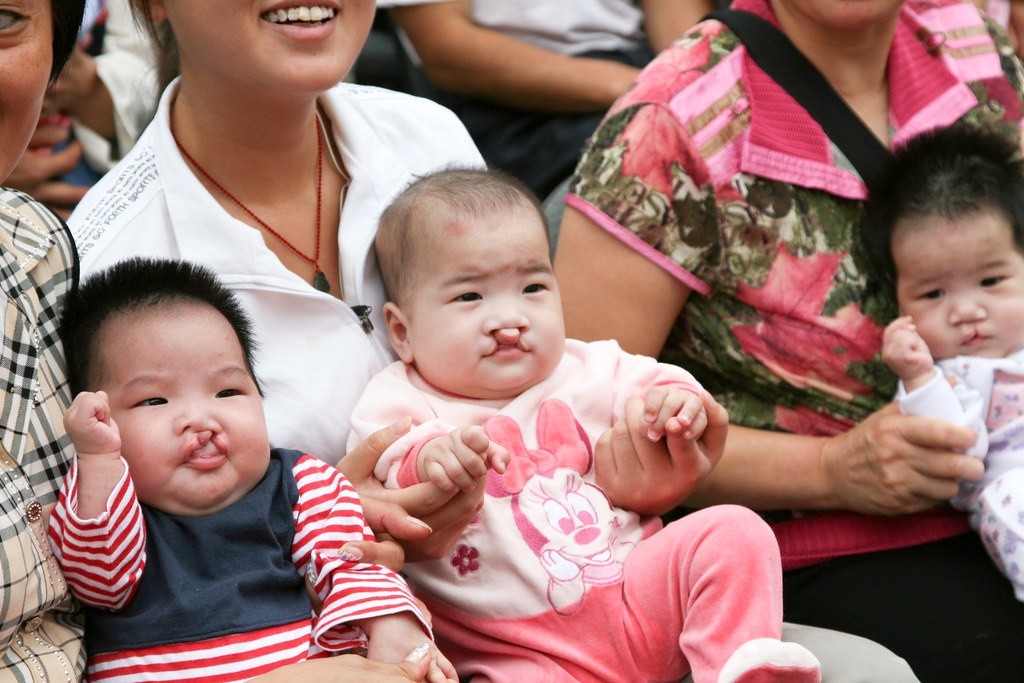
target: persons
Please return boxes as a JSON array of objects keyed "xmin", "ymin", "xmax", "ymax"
[
  {"xmin": 4, "ymin": 0, "xmax": 159, "ymax": 223},
  {"xmin": 48, "ymin": 257, "xmax": 459, "ymax": 683},
  {"xmin": 63, "ymin": 1, "xmax": 920, "ymax": 683},
  {"xmin": 862, "ymin": 124, "xmax": 1024, "ymax": 602},
  {"xmin": 554, "ymin": 2, "xmax": 1024, "ymax": 683},
  {"xmin": 0, "ymin": 1, "xmax": 435, "ymax": 683},
  {"xmin": 345, "ymin": 163, "xmax": 821, "ymax": 683},
  {"xmin": 344, "ymin": 2, "xmax": 732, "ymax": 262}
]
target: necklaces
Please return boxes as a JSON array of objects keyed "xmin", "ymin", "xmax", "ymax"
[{"xmin": 170, "ymin": 115, "xmax": 334, "ymax": 293}]
